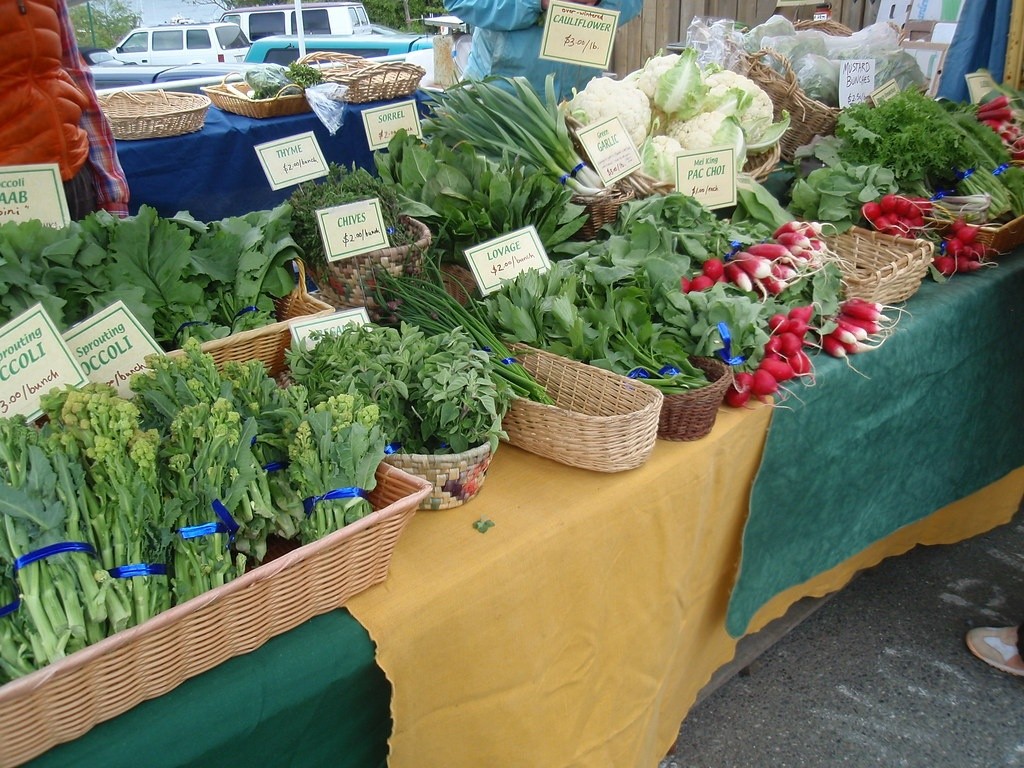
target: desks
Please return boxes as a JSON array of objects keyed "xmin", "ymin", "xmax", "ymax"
[
  {"xmin": 16, "ymin": 251, "xmax": 1024, "ymax": 768},
  {"xmin": 116, "ymin": 91, "xmax": 427, "ymax": 214}
]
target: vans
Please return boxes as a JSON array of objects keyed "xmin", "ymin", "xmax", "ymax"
[
  {"xmin": 243, "ymin": 33, "xmax": 457, "ymax": 76},
  {"xmin": 216, "ymin": 1, "xmax": 371, "ymax": 48}
]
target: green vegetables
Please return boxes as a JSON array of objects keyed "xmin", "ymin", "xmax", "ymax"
[{"xmin": 1, "ymin": 66, "xmax": 1024, "ymax": 688}]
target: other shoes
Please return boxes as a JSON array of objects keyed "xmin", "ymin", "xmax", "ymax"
[{"xmin": 966, "ymin": 627, "xmax": 1024, "ymax": 677}]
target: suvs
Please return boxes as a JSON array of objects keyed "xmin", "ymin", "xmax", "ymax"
[{"xmin": 103, "ymin": 20, "xmax": 252, "ymax": 68}]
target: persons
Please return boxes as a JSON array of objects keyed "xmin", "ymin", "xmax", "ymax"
[
  {"xmin": 0, "ymin": 0, "xmax": 132, "ymax": 220},
  {"xmin": 965, "ymin": 623, "xmax": 1024, "ymax": 677},
  {"xmin": 444, "ymin": 0, "xmax": 641, "ymax": 115}
]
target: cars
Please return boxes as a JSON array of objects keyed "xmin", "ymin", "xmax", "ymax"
[{"xmin": 369, "ymin": 23, "xmax": 408, "ymax": 35}]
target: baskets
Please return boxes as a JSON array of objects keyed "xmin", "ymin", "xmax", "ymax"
[
  {"xmin": 438, "ymin": 262, "xmax": 486, "ymax": 310},
  {"xmin": 924, "ymin": 213, "xmax": 1024, "ymax": 262},
  {"xmin": 559, "ymin": 99, "xmax": 780, "ymax": 220},
  {"xmin": 555, "ymin": 184, "xmax": 636, "ymax": 242},
  {"xmin": 300, "ymin": 215, "xmax": 431, "ymax": 320},
  {"xmin": 201, "ymin": 72, "xmax": 313, "ymax": 119},
  {"xmin": 793, "ymin": 214, "xmax": 934, "ymax": 304},
  {"xmin": 727, "ymin": 17, "xmax": 907, "ymax": 166},
  {"xmin": 166, "ymin": 258, "xmax": 337, "ymax": 376},
  {"xmin": 657, "ymin": 354, "xmax": 733, "ymax": 442},
  {"xmin": 498, "ymin": 336, "xmax": 663, "ymax": 474},
  {"xmin": 96, "ymin": 88, "xmax": 211, "ymax": 140},
  {"xmin": 279, "ymin": 369, "xmax": 497, "ymax": 511},
  {"xmin": 0, "ymin": 460, "xmax": 433, "ymax": 768},
  {"xmin": 296, "ymin": 51, "xmax": 426, "ymax": 102}
]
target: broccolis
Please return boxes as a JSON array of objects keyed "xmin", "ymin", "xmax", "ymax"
[{"xmin": 567, "ymin": 51, "xmax": 790, "ymax": 180}]
